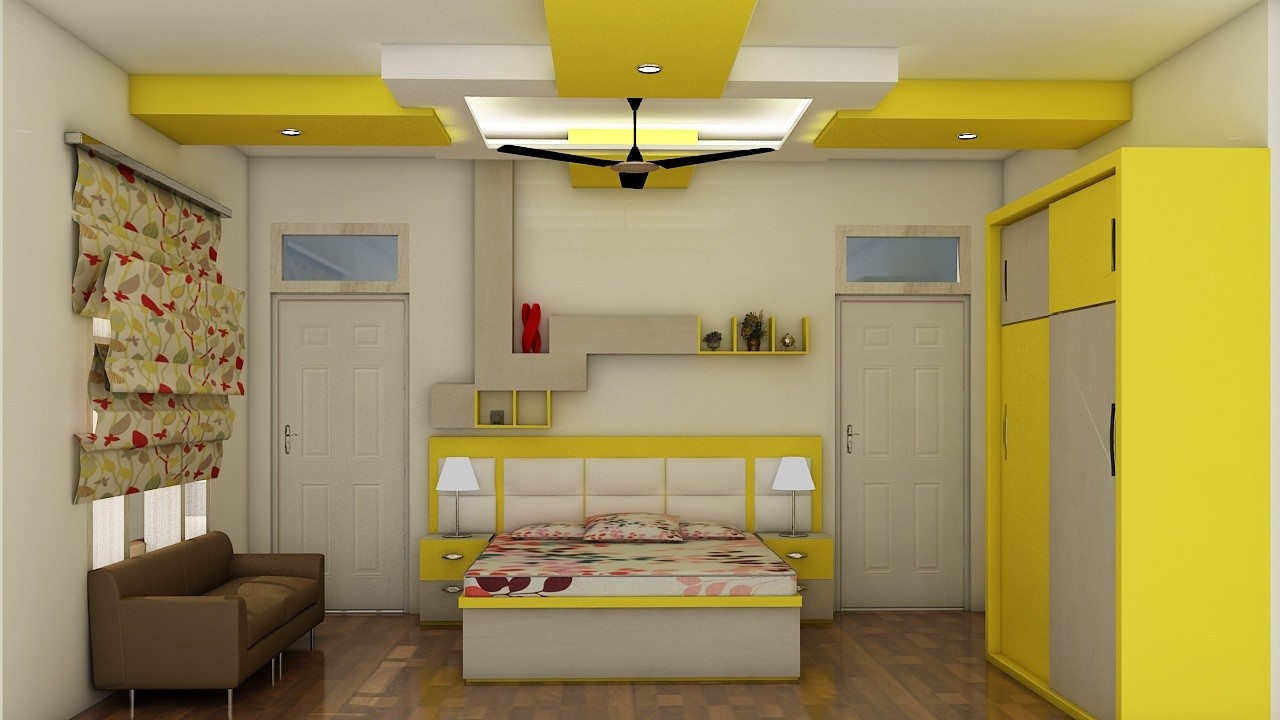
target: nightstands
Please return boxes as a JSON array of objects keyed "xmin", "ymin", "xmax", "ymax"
[
  {"xmin": 755, "ymin": 532, "xmax": 835, "ymax": 623},
  {"xmin": 419, "ymin": 533, "xmax": 495, "ymax": 629}
]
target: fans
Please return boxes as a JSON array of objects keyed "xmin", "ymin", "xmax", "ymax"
[{"xmin": 497, "ymin": 98, "xmax": 775, "ymax": 190}]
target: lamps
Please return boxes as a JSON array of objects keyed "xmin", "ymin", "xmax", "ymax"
[
  {"xmin": 771, "ymin": 457, "xmax": 816, "ymax": 536},
  {"xmin": 436, "ymin": 457, "xmax": 480, "ymax": 537}
]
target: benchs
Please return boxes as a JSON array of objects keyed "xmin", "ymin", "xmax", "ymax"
[{"xmin": 88, "ymin": 531, "xmax": 326, "ymax": 720}]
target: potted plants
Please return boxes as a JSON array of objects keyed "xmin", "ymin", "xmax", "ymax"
[
  {"xmin": 739, "ymin": 310, "xmax": 768, "ymax": 351},
  {"xmin": 704, "ymin": 331, "xmax": 722, "ymax": 351}
]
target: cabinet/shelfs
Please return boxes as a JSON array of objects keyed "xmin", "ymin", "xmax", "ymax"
[
  {"xmin": 984, "ymin": 146, "xmax": 1271, "ymax": 720},
  {"xmin": 429, "ymin": 314, "xmax": 812, "ymax": 428}
]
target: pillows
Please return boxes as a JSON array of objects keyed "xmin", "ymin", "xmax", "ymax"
[
  {"xmin": 680, "ymin": 521, "xmax": 745, "ymax": 539},
  {"xmin": 512, "ymin": 521, "xmax": 585, "ymax": 540},
  {"xmin": 585, "ymin": 512, "xmax": 683, "ymax": 542}
]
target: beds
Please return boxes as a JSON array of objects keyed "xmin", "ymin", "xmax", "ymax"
[{"xmin": 458, "ymin": 519, "xmax": 803, "ymax": 682}]
